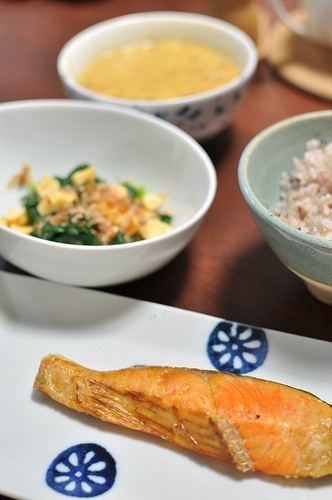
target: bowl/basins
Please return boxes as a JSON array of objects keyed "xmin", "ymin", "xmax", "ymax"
[
  {"xmin": 59, "ymin": 11, "xmax": 260, "ymax": 139},
  {"xmin": 269, "ymin": 0, "xmax": 332, "ymax": 47},
  {"xmin": 0, "ymin": 100, "xmax": 217, "ymax": 288},
  {"xmin": 238, "ymin": 110, "xmax": 332, "ymax": 305}
]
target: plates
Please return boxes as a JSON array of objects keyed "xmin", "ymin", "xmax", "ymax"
[
  {"xmin": 1, "ymin": 270, "xmax": 331, "ymax": 500},
  {"xmin": 257, "ymin": 0, "xmax": 332, "ymax": 101}
]
